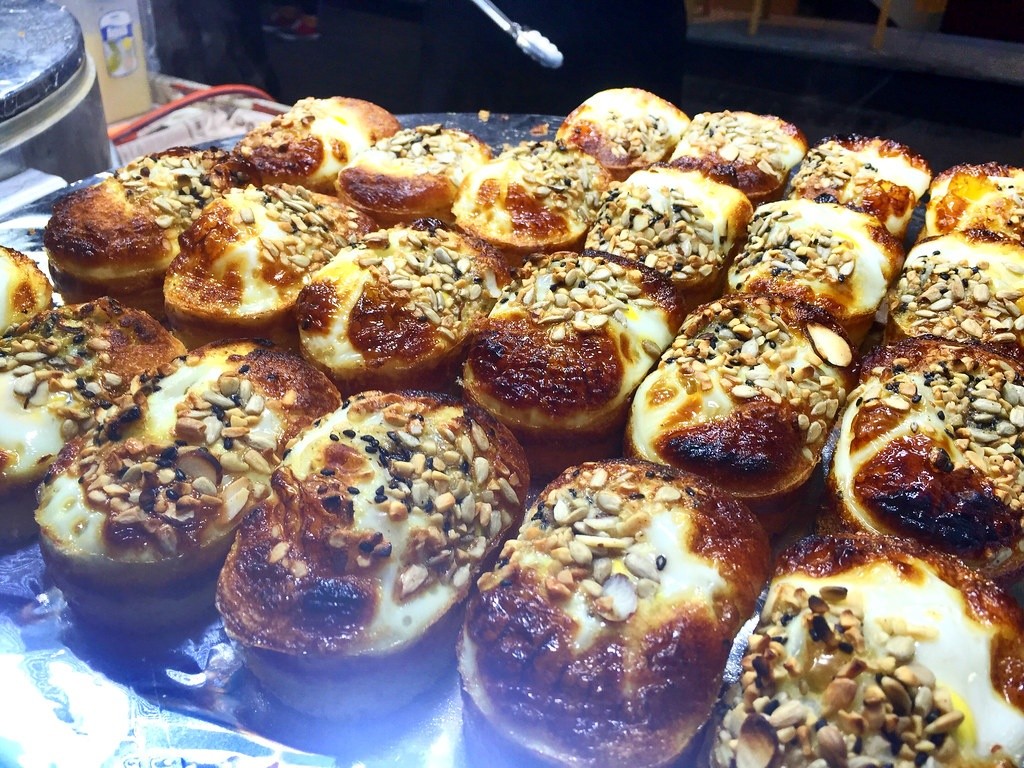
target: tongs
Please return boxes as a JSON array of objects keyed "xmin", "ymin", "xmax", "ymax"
[{"xmin": 472, "ymin": 0, "xmax": 564, "ymax": 70}]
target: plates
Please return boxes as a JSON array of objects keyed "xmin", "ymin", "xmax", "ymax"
[{"xmin": 1, "ymin": 111, "xmax": 768, "ymax": 768}]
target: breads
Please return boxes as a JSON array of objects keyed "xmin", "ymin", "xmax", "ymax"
[{"xmin": 0, "ymin": 85, "xmax": 1024, "ymax": 768}]
